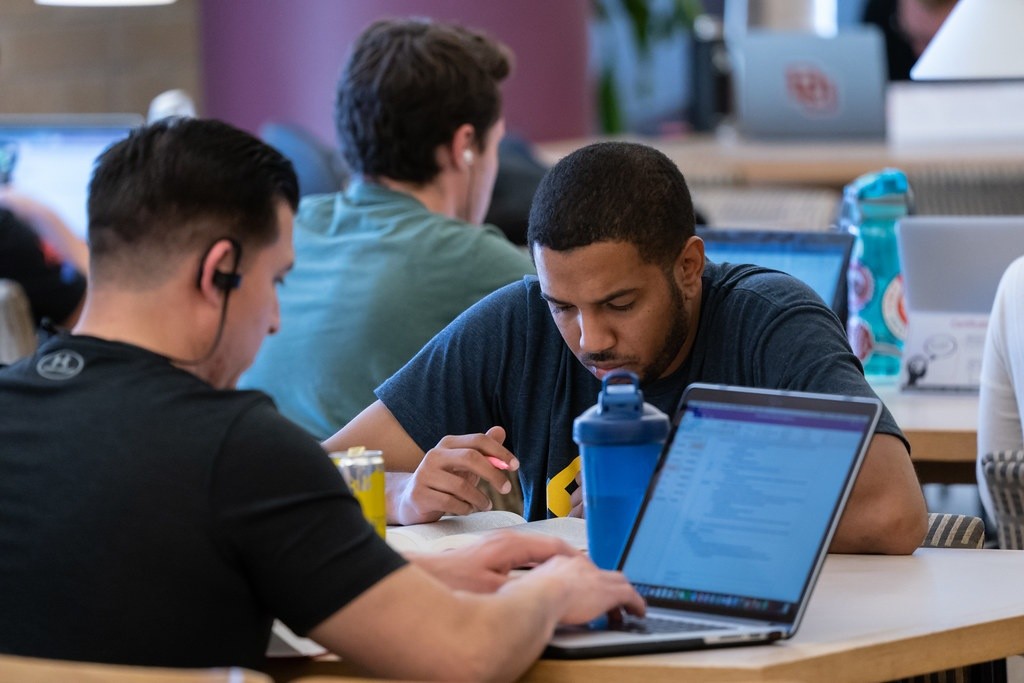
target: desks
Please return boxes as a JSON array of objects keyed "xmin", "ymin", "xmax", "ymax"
[
  {"xmin": 873, "ymin": 380, "xmax": 980, "ymax": 487},
  {"xmin": 532, "ymin": 134, "xmax": 1024, "ymax": 217},
  {"xmin": 265, "ymin": 547, "xmax": 1023, "ymax": 682}
]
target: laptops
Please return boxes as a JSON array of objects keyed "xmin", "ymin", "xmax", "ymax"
[
  {"xmin": 895, "ymin": 217, "xmax": 1024, "ymax": 390},
  {"xmin": 541, "ymin": 382, "xmax": 885, "ymax": 657},
  {"xmin": 0, "ymin": 113, "xmax": 146, "ymax": 248},
  {"xmin": 729, "ymin": 26, "xmax": 890, "ymax": 140}
]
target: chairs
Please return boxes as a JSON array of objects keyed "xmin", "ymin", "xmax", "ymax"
[{"xmin": 896, "ymin": 450, "xmax": 1024, "ymax": 683}]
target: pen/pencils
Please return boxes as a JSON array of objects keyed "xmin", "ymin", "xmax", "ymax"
[{"xmin": 486, "ymin": 454, "xmax": 511, "ymax": 472}]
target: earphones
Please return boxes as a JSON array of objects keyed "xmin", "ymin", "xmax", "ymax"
[
  {"xmin": 462, "ymin": 149, "xmax": 475, "ymax": 166},
  {"xmin": 212, "ymin": 268, "xmax": 240, "ymax": 293}
]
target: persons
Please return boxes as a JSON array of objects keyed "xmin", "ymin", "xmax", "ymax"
[
  {"xmin": 858, "ymin": 0, "xmax": 960, "ymax": 82},
  {"xmin": 0, "ymin": 112, "xmax": 929, "ymax": 683},
  {"xmin": 974, "ymin": 253, "xmax": 1024, "ymax": 551},
  {"xmin": 235, "ymin": 14, "xmax": 535, "ymax": 442}
]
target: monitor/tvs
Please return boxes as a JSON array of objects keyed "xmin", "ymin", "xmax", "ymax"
[{"xmin": 693, "ymin": 228, "xmax": 858, "ymax": 345}]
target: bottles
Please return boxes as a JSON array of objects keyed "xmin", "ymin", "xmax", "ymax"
[
  {"xmin": 840, "ymin": 167, "xmax": 913, "ymax": 383},
  {"xmin": 329, "ymin": 446, "xmax": 387, "ymax": 542},
  {"xmin": 573, "ymin": 367, "xmax": 669, "ymax": 572}
]
